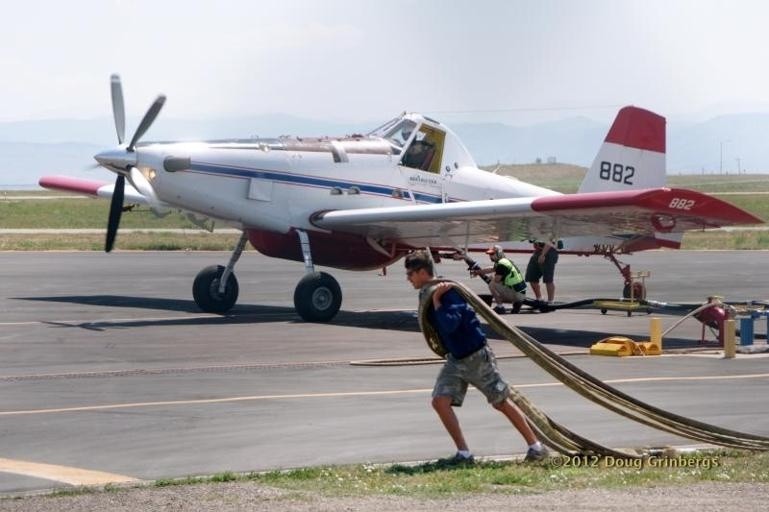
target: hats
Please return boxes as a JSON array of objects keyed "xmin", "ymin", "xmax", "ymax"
[{"xmin": 485, "ymin": 245, "xmax": 503, "ymax": 259}]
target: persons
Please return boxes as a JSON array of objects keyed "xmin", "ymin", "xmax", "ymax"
[
  {"xmin": 403, "ymin": 250, "xmax": 549, "ymax": 467},
  {"xmin": 523, "ymin": 241, "xmax": 563, "ymax": 313},
  {"xmin": 468, "ymin": 247, "xmax": 529, "ymax": 313},
  {"xmin": 395, "ymin": 131, "xmax": 425, "ymax": 168}
]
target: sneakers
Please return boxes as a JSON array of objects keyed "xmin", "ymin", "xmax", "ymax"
[
  {"xmin": 491, "ymin": 301, "xmax": 556, "ymax": 315},
  {"xmin": 439, "ymin": 450, "xmax": 476, "ymax": 466},
  {"xmin": 521, "ymin": 444, "xmax": 550, "ymax": 466}
]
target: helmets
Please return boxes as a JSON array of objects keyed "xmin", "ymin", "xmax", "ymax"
[{"xmin": 401, "ymin": 127, "xmax": 419, "ymax": 142}]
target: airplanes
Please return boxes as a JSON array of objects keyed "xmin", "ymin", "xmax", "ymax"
[{"xmin": 39, "ymin": 75, "xmax": 766, "ymax": 322}]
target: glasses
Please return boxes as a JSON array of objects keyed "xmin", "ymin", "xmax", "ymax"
[{"xmin": 406, "ymin": 269, "xmax": 415, "ymax": 276}]
